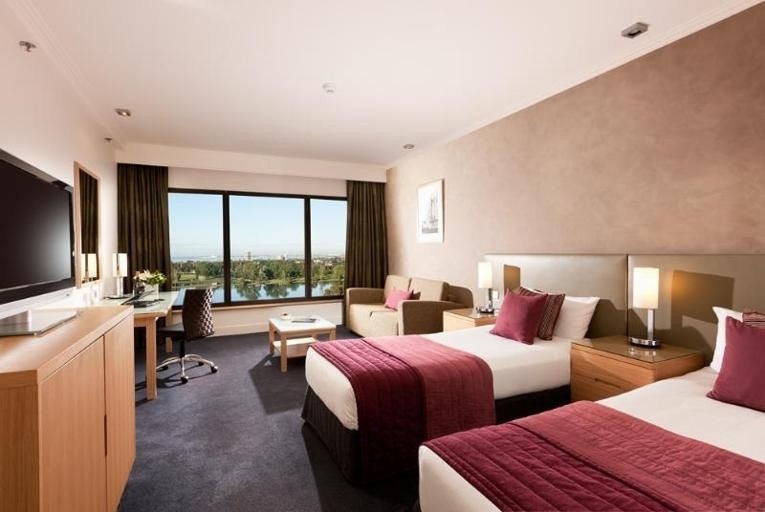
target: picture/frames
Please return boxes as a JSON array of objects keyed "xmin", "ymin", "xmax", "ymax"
[{"xmin": 417, "ymin": 176, "xmax": 447, "ymax": 244}]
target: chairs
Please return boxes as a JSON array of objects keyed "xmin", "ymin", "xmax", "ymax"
[{"xmin": 159, "ymin": 285, "xmax": 219, "ymax": 381}]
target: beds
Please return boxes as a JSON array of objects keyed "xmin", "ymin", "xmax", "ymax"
[
  {"xmin": 296, "ymin": 248, "xmax": 627, "ymax": 486},
  {"xmin": 415, "ymin": 253, "xmax": 764, "ymax": 510}
]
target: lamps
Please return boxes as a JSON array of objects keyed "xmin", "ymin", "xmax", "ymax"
[
  {"xmin": 630, "ymin": 265, "xmax": 664, "ymax": 348},
  {"xmin": 476, "ymin": 260, "xmax": 505, "ymax": 315},
  {"xmin": 112, "ymin": 252, "xmax": 128, "ymax": 294},
  {"xmin": 81, "ymin": 251, "xmax": 98, "ymax": 283}
]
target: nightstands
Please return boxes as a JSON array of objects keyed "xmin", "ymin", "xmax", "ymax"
[
  {"xmin": 561, "ymin": 334, "xmax": 704, "ymax": 403},
  {"xmin": 440, "ymin": 304, "xmax": 502, "ymax": 332}
]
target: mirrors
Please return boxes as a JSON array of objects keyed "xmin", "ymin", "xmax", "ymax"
[{"xmin": 74, "ymin": 159, "xmax": 104, "ymax": 288}]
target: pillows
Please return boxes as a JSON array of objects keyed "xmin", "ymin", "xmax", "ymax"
[
  {"xmin": 742, "ymin": 310, "xmax": 764, "ymax": 329},
  {"xmin": 708, "ymin": 313, "xmax": 764, "ymax": 417},
  {"xmin": 491, "ymin": 286, "xmax": 548, "ymax": 345},
  {"xmin": 711, "ymin": 305, "xmax": 744, "ymax": 376},
  {"xmin": 383, "ymin": 284, "xmax": 415, "ymax": 311},
  {"xmin": 514, "ymin": 287, "xmax": 566, "ymax": 341},
  {"xmin": 544, "ymin": 291, "xmax": 601, "ymax": 343}
]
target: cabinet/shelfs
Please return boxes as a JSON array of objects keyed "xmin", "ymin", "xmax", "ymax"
[{"xmin": 0, "ymin": 302, "xmax": 143, "ymax": 512}]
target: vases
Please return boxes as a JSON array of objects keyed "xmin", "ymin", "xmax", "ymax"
[{"xmin": 144, "ymin": 283, "xmax": 160, "ymax": 297}]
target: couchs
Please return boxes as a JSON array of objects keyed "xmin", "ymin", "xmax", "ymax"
[{"xmin": 344, "ymin": 273, "xmax": 465, "ymax": 336}]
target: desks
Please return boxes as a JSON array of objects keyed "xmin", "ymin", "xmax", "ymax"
[
  {"xmin": 269, "ymin": 313, "xmax": 339, "ymax": 373},
  {"xmin": 98, "ymin": 289, "xmax": 178, "ymax": 402}
]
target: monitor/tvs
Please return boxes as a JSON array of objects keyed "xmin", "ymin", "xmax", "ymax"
[{"xmin": 0, "ymin": 149, "xmax": 78, "ymax": 335}]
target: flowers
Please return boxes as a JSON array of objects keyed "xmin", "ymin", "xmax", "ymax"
[{"xmin": 133, "ymin": 269, "xmax": 168, "ymax": 285}]
